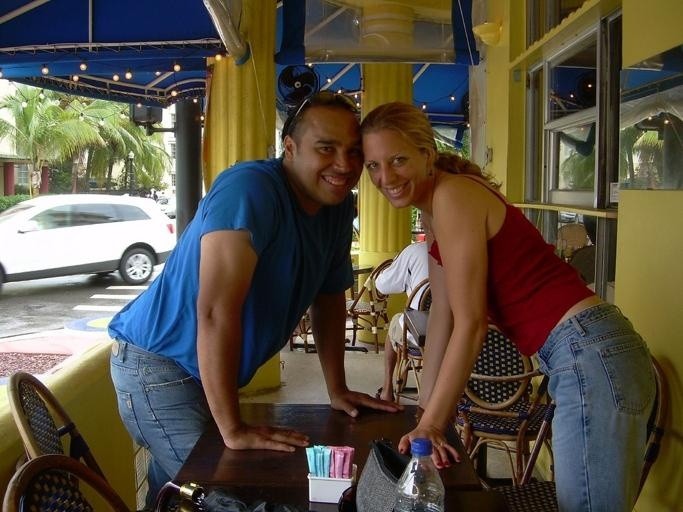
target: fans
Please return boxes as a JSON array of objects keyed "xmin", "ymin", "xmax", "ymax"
[{"xmin": 277, "ymin": 64, "xmax": 318, "ymax": 110}]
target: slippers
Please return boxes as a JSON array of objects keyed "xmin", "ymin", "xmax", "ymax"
[{"xmin": 375, "ymin": 387, "xmax": 395, "ymax": 401}]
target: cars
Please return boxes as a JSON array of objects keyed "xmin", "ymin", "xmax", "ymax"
[{"xmin": 156, "ymin": 197, "xmax": 175, "ymax": 217}]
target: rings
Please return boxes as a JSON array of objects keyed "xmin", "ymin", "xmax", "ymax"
[{"xmin": 436, "ymin": 439, "xmax": 444, "ymax": 449}]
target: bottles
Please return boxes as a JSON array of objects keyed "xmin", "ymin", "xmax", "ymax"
[{"xmin": 398, "ymin": 440, "xmax": 445, "ymax": 512}]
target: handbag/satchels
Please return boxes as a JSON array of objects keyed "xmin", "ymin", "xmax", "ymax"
[{"xmin": 356, "ymin": 440, "xmax": 411, "ymax": 512}]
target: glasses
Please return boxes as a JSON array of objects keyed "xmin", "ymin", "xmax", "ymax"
[{"xmin": 288, "ymin": 93, "xmax": 357, "ymax": 132}]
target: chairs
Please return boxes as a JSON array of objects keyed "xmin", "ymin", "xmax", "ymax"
[
  {"xmin": 8, "ymin": 372, "xmax": 109, "ymax": 494},
  {"xmin": 557, "ymin": 223, "xmax": 587, "ymax": 258},
  {"xmin": 491, "ymin": 354, "xmax": 670, "ymax": 512},
  {"xmin": 457, "ymin": 325, "xmax": 548, "ymax": 485},
  {"xmin": 567, "ymin": 245, "xmax": 614, "ymax": 285},
  {"xmin": 290, "ymin": 306, "xmax": 367, "ymax": 353},
  {"xmin": 2, "ymin": 454, "xmax": 134, "ymax": 511},
  {"xmin": 395, "ymin": 277, "xmax": 432, "ymax": 406},
  {"xmin": 345, "ymin": 259, "xmax": 396, "ymax": 354}
]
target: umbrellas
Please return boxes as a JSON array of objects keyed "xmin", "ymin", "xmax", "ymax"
[{"xmin": 176, "ymin": 479, "xmax": 299, "ymax": 512}]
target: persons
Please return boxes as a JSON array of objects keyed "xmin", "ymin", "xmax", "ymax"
[
  {"xmin": 374, "ymin": 238, "xmax": 431, "ymax": 405},
  {"xmin": 359, "ymin": 101, "xmax": 660, "ymax": 512},
  {"xmin": 105, "ymin": 88, "xmax": 406, "ymax": 512}
]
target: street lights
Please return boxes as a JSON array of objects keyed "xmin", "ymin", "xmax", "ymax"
[{"xmin": 126, "ymin": 151, "xmax": 133, "ymax": 193}]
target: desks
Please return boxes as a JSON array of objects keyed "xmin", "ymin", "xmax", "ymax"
[
  {"xmin": 402, "ymin": 310, "xmax": 429, "ymax": 348},
  {"xmin": 156, "ymin": 403, "xmax": 496, "ymax": 512},
  {"xmin": 350, "ymin": 264, "xmax": 373, "ymax": 300}
]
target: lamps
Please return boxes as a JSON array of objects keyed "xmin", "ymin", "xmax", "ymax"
[
  {"xmin": 472, "ymin": 22, "xmax": 500, "ymax": 47},
  {"xmin": 129, "ymin": 102, "xmax": 175, "ymax": 136}
]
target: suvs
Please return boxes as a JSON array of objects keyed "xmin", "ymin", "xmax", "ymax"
[{"xmin": 0, "ymin": 194, "xmax": 174, "ymax": 284}]
